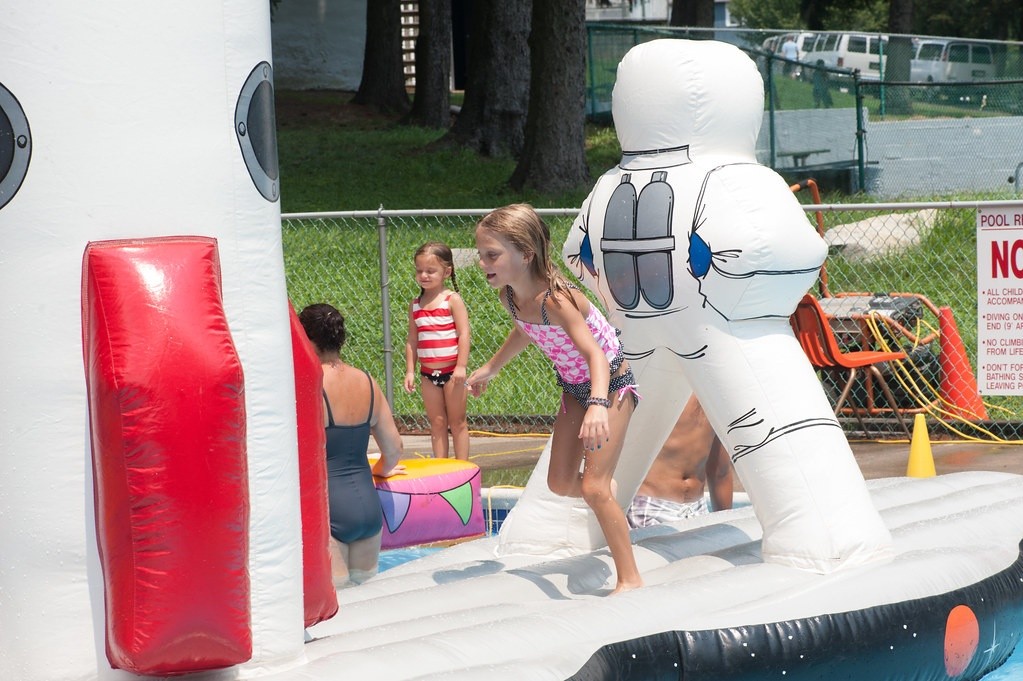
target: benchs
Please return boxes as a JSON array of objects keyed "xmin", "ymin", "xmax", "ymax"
[{"xmin": 777, "ymin": 146, "xmax": 831, "ymax": 167}]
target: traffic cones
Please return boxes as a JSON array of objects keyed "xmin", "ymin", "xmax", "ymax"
[
  {"xmin": 906, "ymin": 412, "xmax": 936, "ymax": 478},
  {"xmin": 932, "ymin": 307, "xmax": 990, "ymax": 419}
]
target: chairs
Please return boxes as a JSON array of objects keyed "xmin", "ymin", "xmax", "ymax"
[{"xmin": 789, "ymin": 293, "xmax": 913, "ymax": 443}]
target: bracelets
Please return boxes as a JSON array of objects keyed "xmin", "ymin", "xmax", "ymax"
[{"xmin": 583, "ymin": 397, "xmax": 611, "ymax": 408}]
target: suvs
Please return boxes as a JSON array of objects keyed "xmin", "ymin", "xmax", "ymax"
[
  {"xmin": 910, "ymin": 39, "xmax": 996, "ymax": 101},
  {"xmin": 757, "ymin": 32, "xmax": 888, "ymax": 85}
]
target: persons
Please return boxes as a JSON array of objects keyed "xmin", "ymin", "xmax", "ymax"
[
  {"xmin": 463, "ymin": 201, "xmax": 639, "ymax": 599},
  {"xmin": 404, "ymin": 240, "xmax": 472, "ymax": 461},
  {"xmin": 297, "ymin": 303, "xmax": 408, "ymax": 587},
  {"xmin": 782, "ymin": 34, "xmax": 800, "ymax": 75},
  {"xmin": 631, "ymin": 390, "xmax": 734, "ymax": 525},
  {"xmin": 811, "ymin": 57, "xmax": 833, "ymax": 107}
]
